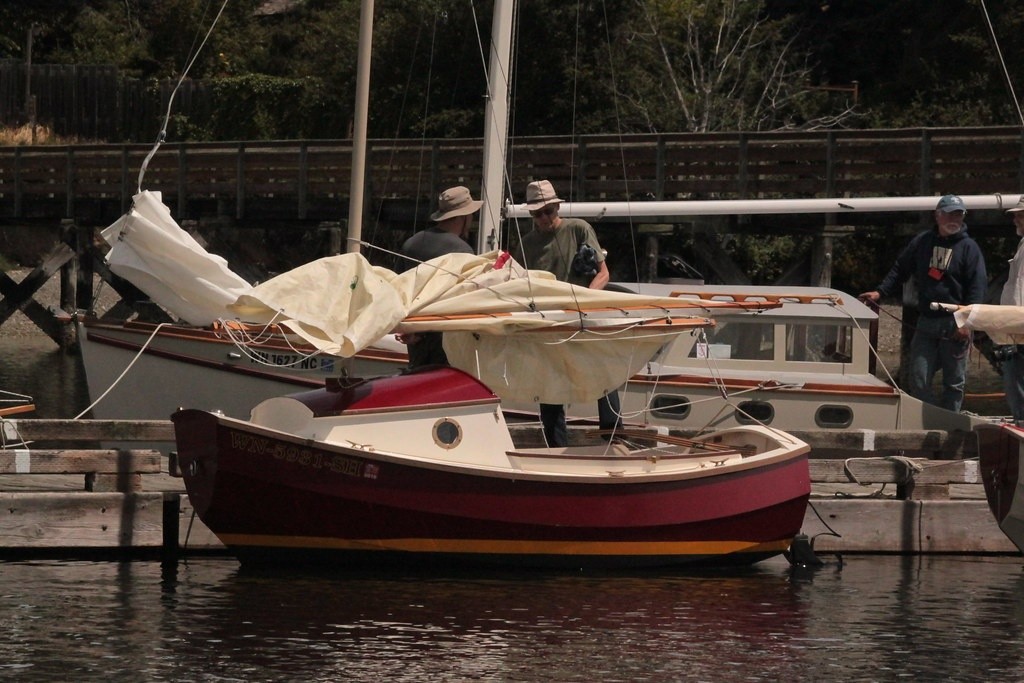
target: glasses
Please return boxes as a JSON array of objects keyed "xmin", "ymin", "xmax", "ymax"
[{"xmin": 530, "ymin": 203, "xmax": 560, "ymax": 218}]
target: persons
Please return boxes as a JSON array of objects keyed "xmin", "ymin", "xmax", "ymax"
[
  {"xmin": 860, "ymin": 195, "xmax": 988, "ymax": 414},
  {"xmin": 992, "ymin": 193, "xmax": 1024, "ymax": 427},
  {"xmin": 513, "ymin": 179, "xmax": 628, "ymax": 448},
  {"xmin": 393, "ymin": 186, "xmax": 485, "ymax": 367}
]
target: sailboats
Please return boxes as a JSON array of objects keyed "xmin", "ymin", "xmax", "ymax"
[{"xmin": 71, "ymin": 0, "xmax": 1015, "ymax": 458}]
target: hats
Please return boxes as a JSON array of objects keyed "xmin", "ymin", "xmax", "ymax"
[
  {"xmin": 520, "ymin": 179, "xmax": 566, "ymax": 211},
  {"xmin": 936, "ymin": 194, "xmax": 968, "ymax": 215},
  {"xmin": 430, "ymin": 185, "xmax": 484, "ymax": 222},
  {"xmin": 1005, "ymin": 193, "xmax": 1024, "ymax": 213}
]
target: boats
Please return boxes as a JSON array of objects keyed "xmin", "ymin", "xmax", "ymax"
[
  {"xmin": 170, "ymin": 365, "xmax": 812, "ymax": 580},
  {"xmin": 975, "ymin": 419, "xmax": 1024, "ymax": 555}
]
